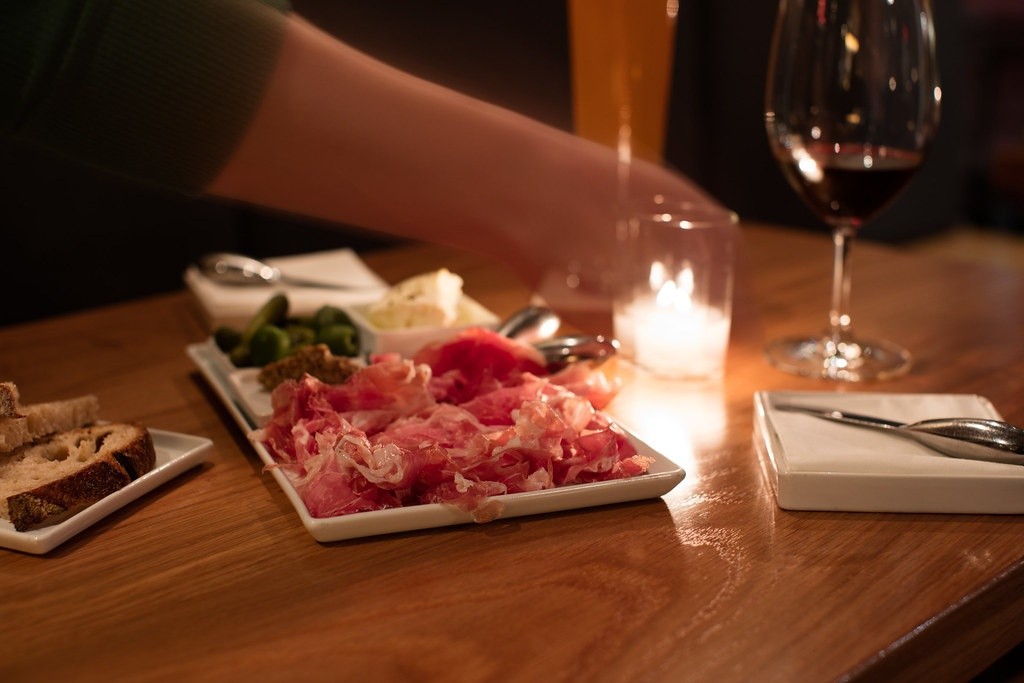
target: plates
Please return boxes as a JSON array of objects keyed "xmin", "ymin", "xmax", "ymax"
[
  {"xmin": 0, "ymin": 419, "xmax": 214, "ymax": 555},
  {"xmin": 185, "ymin": 343, "xmax": 686, "ymax": 544}
]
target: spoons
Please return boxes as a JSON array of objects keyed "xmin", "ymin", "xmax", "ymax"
[
  {"xmin": 501, "ymin": 307, "xmax": 560, "ymax": 340},
  {"xmin": 535, "ymin": 337, "xmax": 617, "ymax": 374},
  {"xmin": 775, "ymin": 401, "xmax": 1024, "ymax": 464},
  {"xmin": 205, "ymin": 253, "xmax": 352, "ymax": 291}
]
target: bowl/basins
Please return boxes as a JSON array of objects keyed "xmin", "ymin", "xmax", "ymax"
[{"xmin": 343, "ymin": 296, "xmax": 499, "ymax": 357}]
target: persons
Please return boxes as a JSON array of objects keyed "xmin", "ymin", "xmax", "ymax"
[{"xmin": 0, "ymin": 0, "xmax": 738, "ymax": 325}]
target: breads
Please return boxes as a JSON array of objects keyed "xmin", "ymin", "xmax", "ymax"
[{"xmin": 0, "ymin": 380, "xmax": 157, "ymax": 531}]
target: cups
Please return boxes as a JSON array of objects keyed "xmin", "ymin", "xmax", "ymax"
[{"xmin": 611, "ymin": 199, "xmax": 739, "ymax": 380}]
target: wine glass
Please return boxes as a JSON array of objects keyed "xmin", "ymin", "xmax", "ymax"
[{"xmin": 758, "ymin": 2, "xmax": 946, "ymax": 386}]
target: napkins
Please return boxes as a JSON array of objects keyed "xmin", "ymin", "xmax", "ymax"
[
  {"xmin": 754, "ymin": 387, "xmax": 1022, "ymax": 517},
  {"xmin": 188, "ymin": 246, "xmax": 390, "ymax": 328}
]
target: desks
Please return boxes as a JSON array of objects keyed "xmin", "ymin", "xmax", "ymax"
[{"xmin": 0, "ymin": 221, "xmax": 1023, "ymax": 683}]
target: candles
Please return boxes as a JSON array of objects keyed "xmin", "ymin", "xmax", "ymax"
[{"xmin": 610, "ymin": 204, "xmax": 739, "ymax": 377}]
